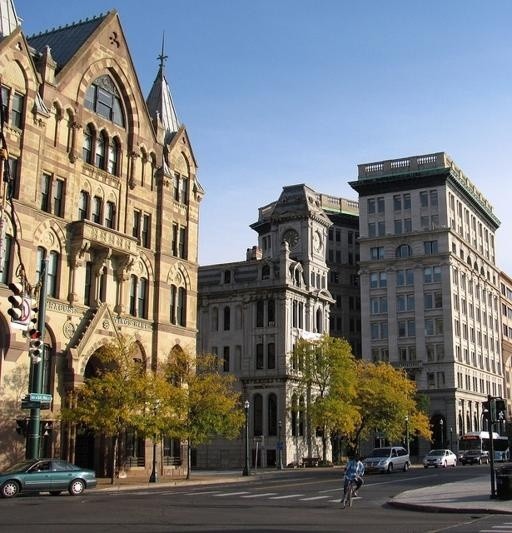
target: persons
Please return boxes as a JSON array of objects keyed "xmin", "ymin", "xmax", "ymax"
[
  {"xmin": 340, "ymin": 454, "xmax": 365, "ymax": 504},
  {"xmin": 33, "ymin": 466, "xmax": 39, "ymax": 472}
]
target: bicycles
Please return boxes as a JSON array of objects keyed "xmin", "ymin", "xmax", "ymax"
[{"xmin": 343, "ymin": 472, "xmax": 359, "ymax": 508}]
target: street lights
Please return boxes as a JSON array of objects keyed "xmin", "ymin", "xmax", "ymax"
[
  {"xmin": 439, "ymin": 417, "xmax": 444, "ymax": 448},
  {"xmin": 147, "ymin": 396, "xmax": 162, "ymax": 482},
  {"xmin": 403, "ymin": 413, "xmax": 412, "ymax": 465},
  {"xmin": 276, "ymin": 417, "xmax": 287, "ymax": 470},
  {"xmin": 240, "ymin": 398, "xmax": 253, "ymax": 475},
  {"xmin": 449, "ymin": 425, "xmax": 454, "ymax": 450}
]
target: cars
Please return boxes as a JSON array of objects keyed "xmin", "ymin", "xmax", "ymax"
[
  {"xmin": 422, "ymin": 446, "xmax": 458, "ymax": 468},
  {"xmin": 362, "ymin": 444, "xmax": 413, "ymax": 472},
  {"xmin": 0, "ymin": 456, "xmax": 97, "ymax": 497},
  {"xmin": 458, "ymin": 448, "xmax": 491, "ymax": 467}
]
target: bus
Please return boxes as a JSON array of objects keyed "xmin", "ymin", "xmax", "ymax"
[{"xmin": 455, "ymin": 427, "xmax": 511, "ymax": 464}]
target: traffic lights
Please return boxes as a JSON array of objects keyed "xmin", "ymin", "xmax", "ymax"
[
  {"xmin": 6, "ymin": 280, "xmax": 24, "ymax": 319},
  {"xmin": 20, "ymin": 301, "xmax": 38, "ymax": 337},
  {"xmin": 39, "ymin": 418, "xmax": 53, "ymax": 439},
  {"xmin": 481, "ymin": 400, "xmax": 490, "ymax": 422},
  {"xmin": 31, "ymin": 340, "xmax": 45, "ymax": 365},
  {"xmin": 27, "ymin": 327, "xmax": 42, "ymax": 358},
  {"xmin": 494, "ymin": 398, "xmax": 509, "ymax": 421},
  {"xmin": 14, "ymin": 417, "xmax": 27, "ymax": 439}
]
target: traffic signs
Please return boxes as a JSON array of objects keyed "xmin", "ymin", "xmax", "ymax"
[
  {"xmin": 40, "ymin": 402, "xmax": 51, "ymax": 409},
  {"xmin": 22, "ymin": 402, "xmax": 39, "ymax": 408},
  {"xmin": 29, "ymin": 393, "xmax": 52, "ymax": 400}
]
